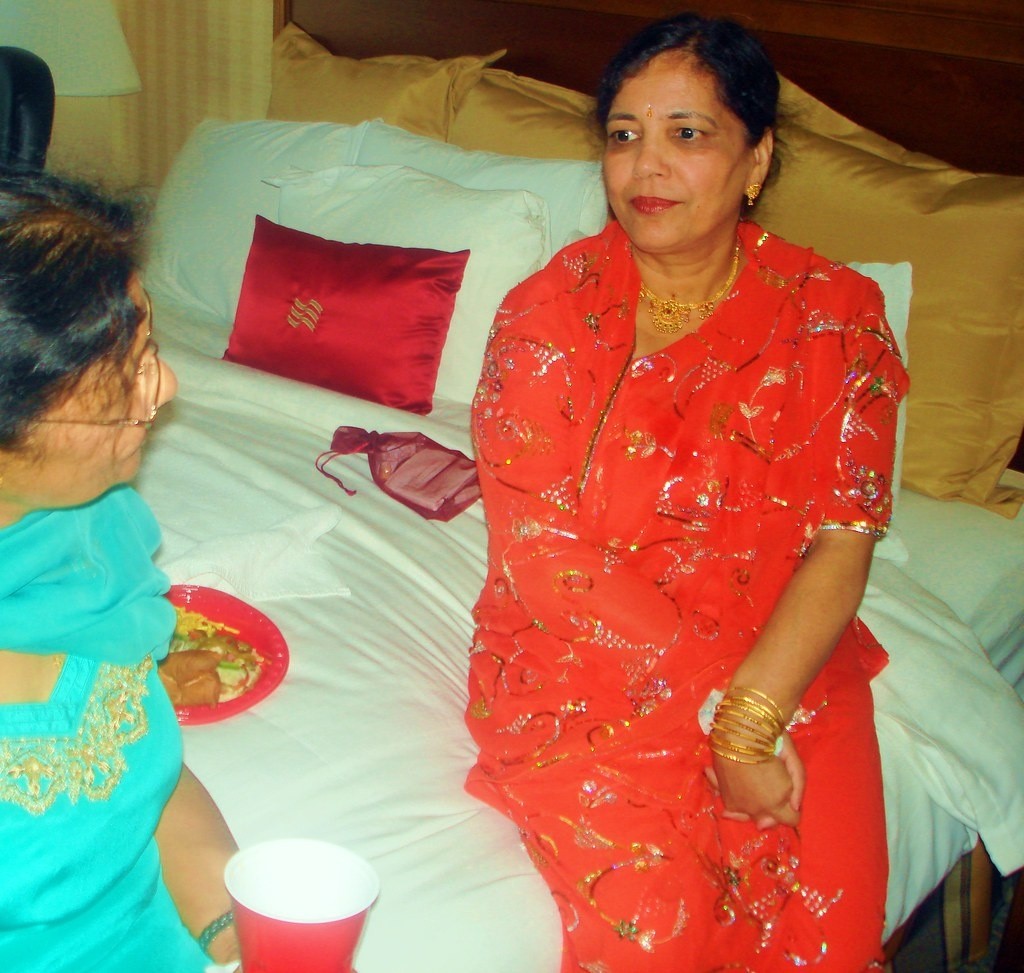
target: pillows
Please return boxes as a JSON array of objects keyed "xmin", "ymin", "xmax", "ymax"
[
  {"xmin": 138, "ymin": 115, "xmax": 366, "ymax": 357},
  {"xmin": 265, "ymin": 22, "xmax": 506, "ymax": 143},
  {"xmin": 565, "ymin": 229, "xmax": 912, "ymax": 566},
  {"xmin": 258, "ymin": 163, "xmax": 553, "ymax": 406},
  {"xmin": 446, "ymin": 70, "xmax": 608, "ymax": 161},
  {"xmin": 223, "ymin": 215, "xmax": 471, "ymax": 416},
  {"xmin": 751, "ymin": 73, "xmax": 1024, "ymax": 520},
  {"xmin": 354, "ymin": 115, "xmax": 608, "ymax": 257}
]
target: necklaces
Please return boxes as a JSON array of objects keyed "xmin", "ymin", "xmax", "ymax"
[{"xmin": 639, "ymin": 237, "xmax": 744, "ymax": 336}]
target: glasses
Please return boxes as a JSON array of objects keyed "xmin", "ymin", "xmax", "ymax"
[{"xmin": 15, "ymin": 289, "xmax": 161, "ymax": 427}]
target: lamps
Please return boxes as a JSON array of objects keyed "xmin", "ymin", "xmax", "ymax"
[{"xmin": 0, "ymin": 0, "xmax": 143, "ymax": 98}]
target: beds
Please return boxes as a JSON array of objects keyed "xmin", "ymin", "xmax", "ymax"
[{"xmin": 0, "ymin": 0, "xmax": 1024, "ymax": 973}]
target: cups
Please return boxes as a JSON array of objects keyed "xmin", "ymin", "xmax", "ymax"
[{"xmin": 222, "ymin": 837, "xmax": 381, "ymax": 973}]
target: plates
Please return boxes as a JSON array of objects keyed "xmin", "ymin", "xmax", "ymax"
[{"xmin": 157, "ymin": 585, "xmax": 289, "ymax": 727}]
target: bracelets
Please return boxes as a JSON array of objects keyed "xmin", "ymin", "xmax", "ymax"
[
  {"xmin": 199, "ymin": 908, "xmax": 232, "ymax": 954},
  {"xmin": 708, "ymin": 686, "xmax": 784, "ymax": 765}
]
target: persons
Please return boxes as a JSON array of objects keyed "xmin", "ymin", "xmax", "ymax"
[
  {"xmin": 464, "ymin": 14, "xmax": 911, "ymax": 973},
  {"xmin": 0, "ymin": 166, "xmax": 246, "ymax": 973}
]
